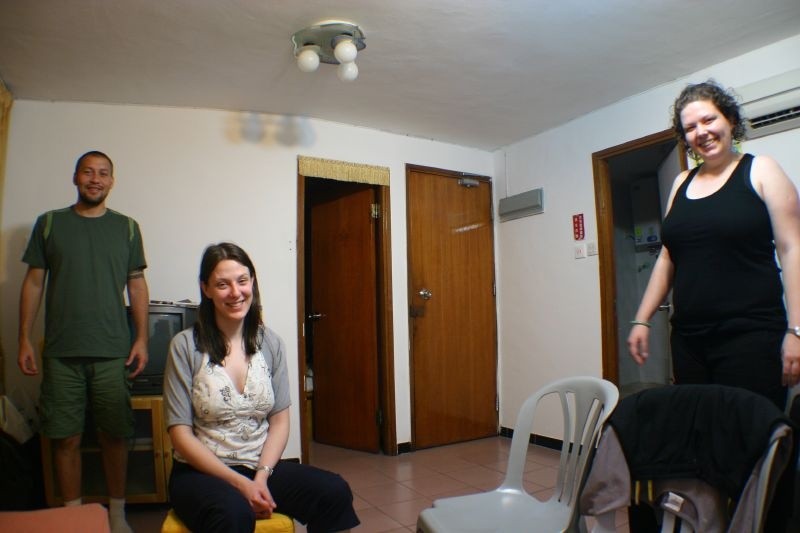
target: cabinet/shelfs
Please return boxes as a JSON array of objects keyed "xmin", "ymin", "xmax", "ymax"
[{"xmin": 40, "ymin": 396, "xmax": 172, "ymax": 508}]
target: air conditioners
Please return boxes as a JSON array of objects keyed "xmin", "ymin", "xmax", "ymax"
[{"xmin": 730, "ymin": 68, "xmax": 800, "ymax": 140}]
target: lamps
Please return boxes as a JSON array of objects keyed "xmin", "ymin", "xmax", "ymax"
[{"xmin": 292, "ymin": 20, "xmax": 367, "ymax": 84}]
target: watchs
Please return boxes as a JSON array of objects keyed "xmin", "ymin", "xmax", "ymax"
[{"xmin": 784, "ymin": 327, "xmax": 800, "ymax": 337}]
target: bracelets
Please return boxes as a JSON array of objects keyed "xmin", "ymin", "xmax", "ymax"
[
  {"xmin": 256, "ymin": 466, "xmax": 274, "ymax": 478},
  {"xmin": 629, "ymin": 321, "xmax": 652, "ymax": 328}
]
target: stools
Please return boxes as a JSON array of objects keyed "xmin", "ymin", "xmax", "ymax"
[{"xmin": 160, "ymin": 507, "xmax": 296, "ymax": 533}]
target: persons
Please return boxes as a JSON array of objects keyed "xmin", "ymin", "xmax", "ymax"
[
  {"xmin": 18, "ymin": 151, "xmax": 150, "ymax": 533},
  {"xmin": 162, "ymin": 242, "xmax": 361, "ymax": 533},
  {"xmin": 627, "ymin": 79, "xmax": 800, "ymax": 533}
]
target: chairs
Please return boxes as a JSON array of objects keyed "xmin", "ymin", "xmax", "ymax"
[
  {"xmin": 576, "ymin": 383, "xmax": 800, "ymax": 533},
  {"xmin": 416, "ymin": 375, "xmax": 619, "ymax": 533}
]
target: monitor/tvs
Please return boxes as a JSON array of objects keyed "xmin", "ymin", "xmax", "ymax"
[{"xmin": 127, "ymin": 306, "xmax": 200, "ymax": 395}]
